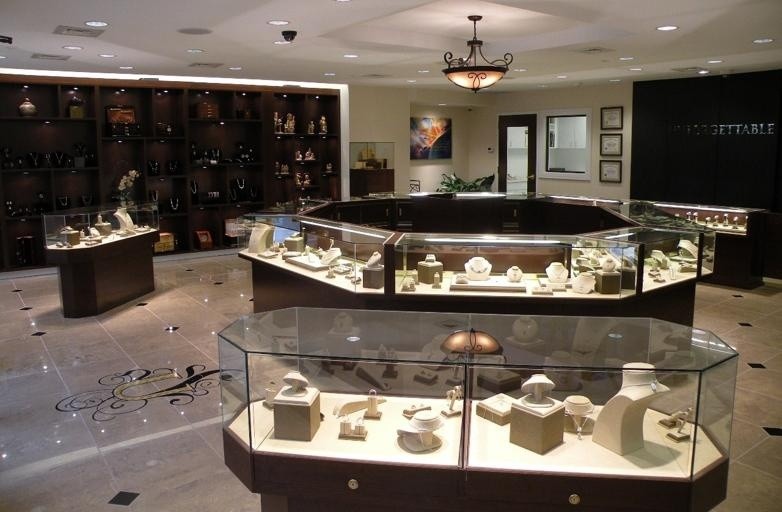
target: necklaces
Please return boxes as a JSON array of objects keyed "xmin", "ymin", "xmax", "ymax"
[
  {"xmin": 550, "ymin": 267, "xmax": 564, "ymax": 280},
  {"xmin": 564, "ymin": 403, "xmax": 594, "ymax": 440},
  {"xmin": 511, "ymin": 269, "xmax": 518, "ymax": 277},
  {"xmin": 605, "ymin": 261, "xmax": 613, "ymax": 267},
  {"xmin": 29, "ymin": 150, "xmax": 93, "ymax": 207},
  {"xmin": 323, "ymin": 251, "xmax": 337, "ymax": 261},
  {"xmin": 621, "ymin": 378, "xmax": 658, "ymax": 392},
  {"xmin": 147, "ymin": 160, "xmax": 245, "ymax": 212},
  {"xmin": 468, "ymin": 260, "xmax": 489, "ymax": 274}
]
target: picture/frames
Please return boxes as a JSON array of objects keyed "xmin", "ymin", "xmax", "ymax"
[{"xmin": 599, "ymin": 106, "xmax": 624, "ymax": 183}]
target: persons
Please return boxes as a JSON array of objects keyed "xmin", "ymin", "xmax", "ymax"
[{"xmin": 275, "ymin": 109, "xmax": 328, "ymax": 188}]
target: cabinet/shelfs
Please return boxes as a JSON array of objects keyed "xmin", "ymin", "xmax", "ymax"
[
  {"xmin": 184, "ymin": 80, "xmax": 341, "ymax": 257},
  {"xmin": 506, "ymin": 117, "xmax": 586, "ymax": 150},
  {"xmin": 0, "ymin": 75, "xmax": 184, "ymax": 276}
]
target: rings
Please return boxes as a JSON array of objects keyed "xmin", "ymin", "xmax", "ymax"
[
  {"xmin": 411, "ymin": 404, "xmax": 417, "ymax": 409},
  {"xmin": 356, "ymin": 417, "xmax": 364, "ymax": 425},
  {"xmin": 343, "ymin": 414, "xmax": 350, "ymax": 422},
  {"xmin": 369, "ymin": 388, "xmax": 377, "ymax": 397}
]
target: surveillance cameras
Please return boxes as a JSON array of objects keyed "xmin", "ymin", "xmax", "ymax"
[{"xmin": 281, "ymin": 31, "xmax": 297, "ymax": 42}]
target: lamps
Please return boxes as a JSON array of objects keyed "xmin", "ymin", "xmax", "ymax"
[{"xmin": 442, "ymin": 15, "xmax": 513, "ymax": 94}]
